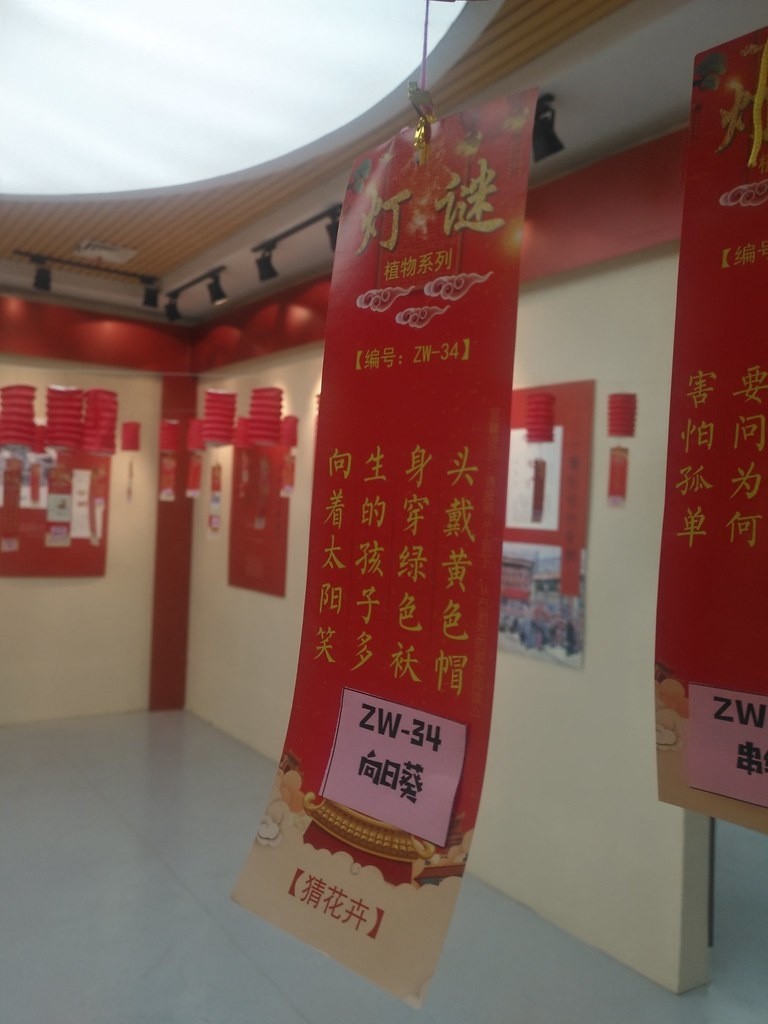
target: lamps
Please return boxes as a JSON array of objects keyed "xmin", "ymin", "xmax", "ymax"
[{"xmin": 11, "ymin": 92, "xmax": 565, "ymax": 324}]
